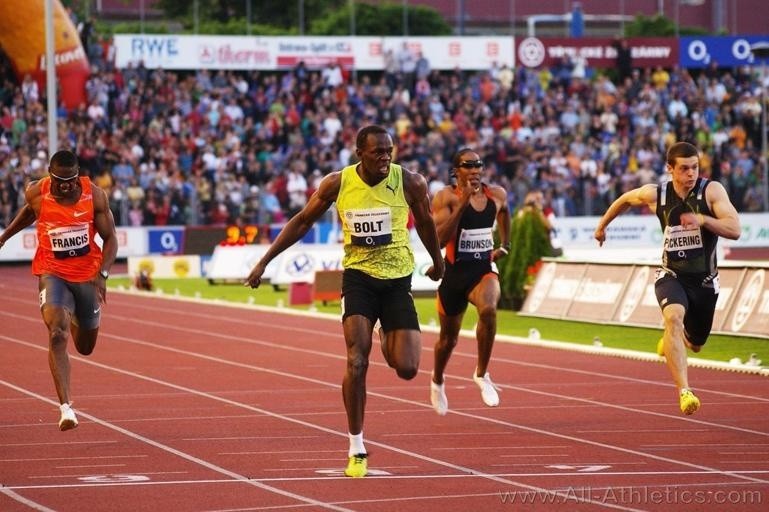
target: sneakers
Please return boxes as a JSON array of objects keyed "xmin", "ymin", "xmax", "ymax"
[
  {"xmin": 473, "ymin": 366, "xmax": 500, "ymax": 406},
  {"xmin": 657, "ymin": 339, "xmax": 664, "ymax": 356},
  {"xmin": 345, "ymin": 453, "xmax": 368, "ymax": 478},
  {"xmin": 59, "ymin": 403, "xmax": 78, "ymax": 432},
  {"xmin": 430, "ymin": 368, "xmax": 449, "ymax": 416},
  {"xmin": 680, "ymin": 389, "xmax": 700, "ymax": 416}
]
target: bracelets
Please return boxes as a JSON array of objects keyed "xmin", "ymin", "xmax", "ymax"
[{"xmin": 496, "ymin": 241, "xmax": 512, "ymax": 255}]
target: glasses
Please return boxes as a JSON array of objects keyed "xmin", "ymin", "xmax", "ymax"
[
  {"xmin": 456, "ymin": 160, "xmax": 483, "ymax": 168},
  {"xmin": 50, "ymin": 168, "xmax": 80, "ymax": 182}
]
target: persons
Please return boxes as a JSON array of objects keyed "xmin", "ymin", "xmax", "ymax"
[
  {"xmin": 1, "ymin": 3, "xmax": 768, "ymax": 226},
  {"xmin": 593, "ymin": 141, "xmax": 740, "ymax": 416},
  {"xmin": 243, "ymin": 126, "xmax": 447, "ymax": 478},
  {"xmin": 428, "ymin": 146, "xmax": 513, "ymax": 417},
  {"xmin": 1, "ymin": 149, "xmax": 119, "ymax": 433}
]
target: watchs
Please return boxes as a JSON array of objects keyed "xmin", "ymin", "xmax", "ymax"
[{"xmin": 99, "ymin": 270, "xmax": 110, "ymax": 281}]
